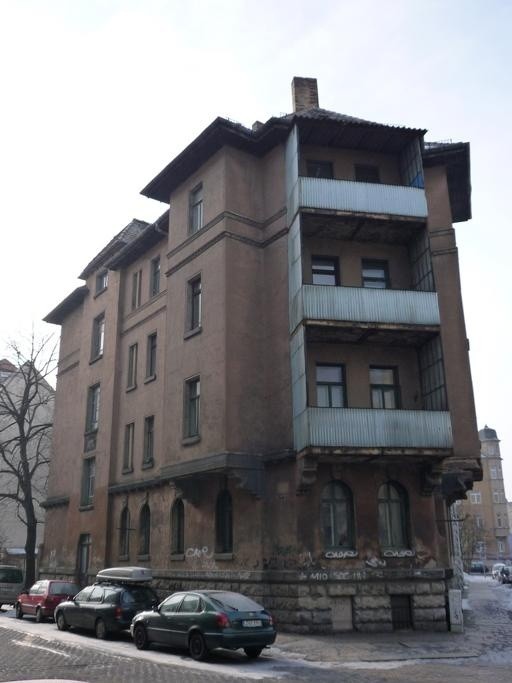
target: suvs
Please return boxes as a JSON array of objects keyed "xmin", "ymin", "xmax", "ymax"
[
  {"xmin": 52, "ymin": 565, "xmax": 161, "ymax": 640},
  {"xmin": 15, "ymin": 578, "xmax": 79, "ymax": 623}
]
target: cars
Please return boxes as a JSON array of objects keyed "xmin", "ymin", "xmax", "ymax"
[
  {"xmin": 463, "ymin": 560, "xmax": 512, "ymax": 584},
  {"xmin": 128, "ymin": 587, "xmax": 284, "ymax": 661}
]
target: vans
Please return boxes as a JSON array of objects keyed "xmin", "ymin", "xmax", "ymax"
[{"xmin": 0, "ymin": 564, "xmax": 23, "ymax": 609}]
